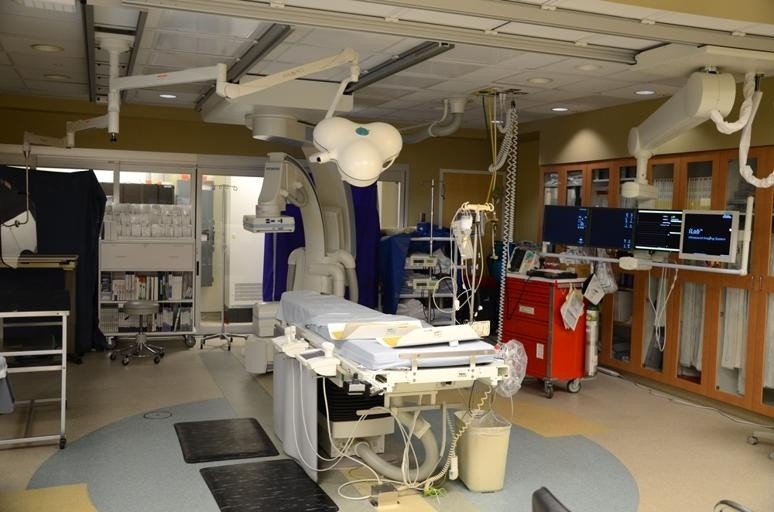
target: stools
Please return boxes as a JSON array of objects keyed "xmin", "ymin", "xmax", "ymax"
[{"xmin": 109, "ymin": 296, "xmax": 169, "ymax": 365}]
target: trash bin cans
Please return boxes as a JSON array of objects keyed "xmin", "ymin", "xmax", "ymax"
[{"xmin": 455, "ymin": 410, "xmax": 512, "ymax": 493}]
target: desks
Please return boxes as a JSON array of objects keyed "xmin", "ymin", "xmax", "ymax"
[{"xmin": 587, "ymin": 158, "xmax": 639, "ymax": 374}]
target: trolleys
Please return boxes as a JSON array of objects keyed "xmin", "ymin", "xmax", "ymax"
[{"xmin": 500, "ymin": 269, "xmax": 603, "ymax": 399}]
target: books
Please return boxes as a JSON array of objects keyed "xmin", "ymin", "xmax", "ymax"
[{"xmin": 100, "ymin": 272, "xmax": 192, "ymax": 334}]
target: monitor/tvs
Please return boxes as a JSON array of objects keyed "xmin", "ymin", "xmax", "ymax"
[
  {"xmin": 586, "ymin": 208, "xmax": 637, "ymax": 249},
  {"xmin": 678, "ymin": 210, "xmax": 739, "ymax": 264},
  {"xmin": 635, "ymin": 209, "xmax": 683, "ymax": 251},
  {"xmin": 542, "ymin": 205, "xmax": 588, "ymax": 246}
]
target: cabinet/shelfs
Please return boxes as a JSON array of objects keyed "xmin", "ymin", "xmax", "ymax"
[
  {"xmin": 707, "ymin": 146, "xmax": 774, "ymax": 418},
  {"xmin": 539, "ymin": 164, "xmax": 587, "ymax": 269},
  {"xmin": 637, "ymin": 150, "xmax": 721, "ymax": 402},
  {"xmin": 101, "ymin": 202, "xmax": 199, "ymax": 337},
  {"xmin": 502, "ymin": 270, "xmax": 591, "ymax": 395}
]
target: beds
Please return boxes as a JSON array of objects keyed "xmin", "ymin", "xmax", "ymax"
[{"xmin": 270, "ymin": 287, "xmax": 513, "ymax": 487}]
